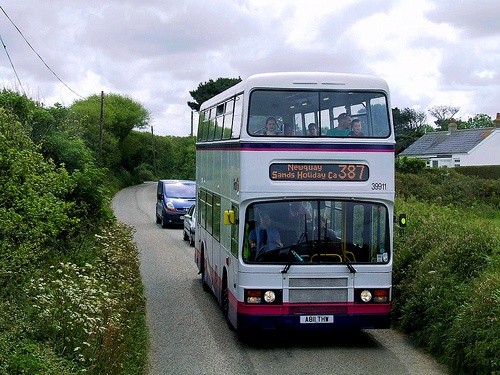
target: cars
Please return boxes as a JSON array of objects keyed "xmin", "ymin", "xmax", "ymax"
[{"xmin": 181, "ymin": 203, "xmax": 196, "ymax": 246}]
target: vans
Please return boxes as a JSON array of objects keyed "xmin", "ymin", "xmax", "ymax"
[{"xmin": 156, "ymin": 180, "xmax": 196, "ymax": 228}]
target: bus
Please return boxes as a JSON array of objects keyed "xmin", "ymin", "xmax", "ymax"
[{"xmin": 194, "ymin": 72, "xmax": 396, "ymax": 336}]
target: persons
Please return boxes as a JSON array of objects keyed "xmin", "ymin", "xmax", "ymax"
[
  {"xmin": 313, "ymin": 216, "xmax": 337, "ymax": 240},
  {"xmin": 307, "ymin": 123, "xmax": 320, "ymax": 136},
  {"xmin": 289, "ymin": 201, "xmax": 312, "ymax": 221},
  {"xmin": 325, "ymin": 114, "xmax": 364, "ymax": 136},
  {"xmin": 248, "ymin": 212, "xmax": 283, "ymax": 262},
  {"xmin": 284, "ymin": 124, "xmax": 295, "ymax": 136},
  {"xmin": 261, "ymin": 116, "xmax": 280, "ymax": 136}
]
